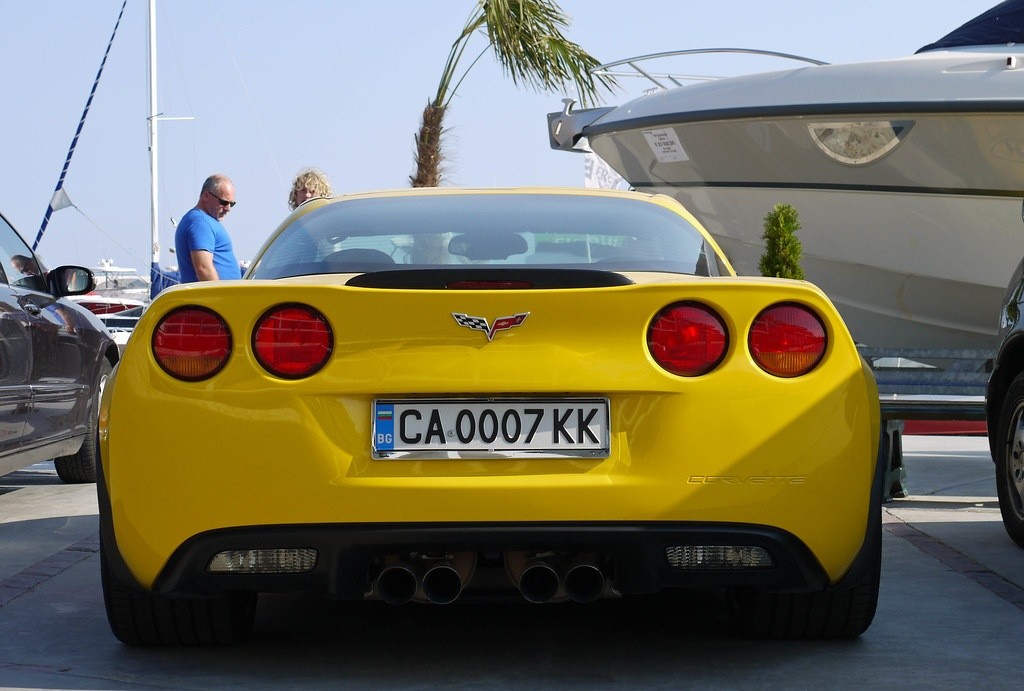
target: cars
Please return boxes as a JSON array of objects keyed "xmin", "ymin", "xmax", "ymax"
[{"xmin": 0, "ymin": 211, "xmax": 123, "ymax": 486}]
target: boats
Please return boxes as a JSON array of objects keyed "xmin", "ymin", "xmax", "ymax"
[
  {"xmin": 75, "ymin": 254, "xmax": 158, "ymax": 331},
  {"xmin": 546, "ymin": 0, "xmax": 1024, "ymax": 349}
]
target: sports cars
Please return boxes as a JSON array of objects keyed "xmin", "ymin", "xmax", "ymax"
[{"xmin": 96, "ymin": 187, "xmax": 883, "ymax": 644}]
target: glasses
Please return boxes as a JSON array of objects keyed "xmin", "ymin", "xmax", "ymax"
[{"xmin": 208, "ymin": 191, "xmax": 236, "ymax": 207}]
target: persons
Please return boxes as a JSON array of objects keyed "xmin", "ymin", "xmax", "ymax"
[
  {"xmin": 10, "ymin": 254, "xmax": 39, "ymax": 276},
  {"xmin": 175, "ymin": 173, "xmax": 242, "ymax": 284},
  {"xmin": 287, "ymin": 166, "xmax": 341, "ymax": 261}
]
